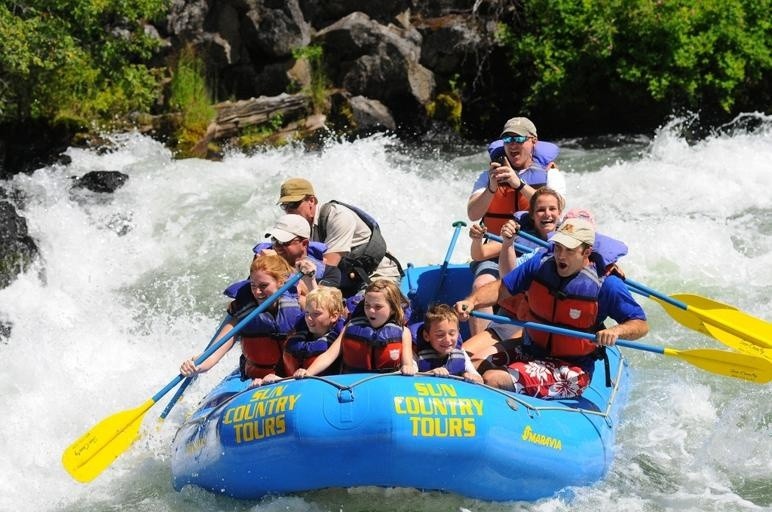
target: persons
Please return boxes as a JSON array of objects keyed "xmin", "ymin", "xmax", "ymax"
[
  {"xmin": 258, "ymin": 216, "xmax": 341, "ymax": 292},
  {"xmin": 294, "ymin": 280, "xmax": 413, "ymax": 379},
  {"xmin": 467, "ymin": 117, "xmax": 565, "ymax": 338},
  {"xmin": 179, "ymin": 257, "xmax": 318, "ymax": 381},
  {"xmin": 498, "ymin": 210, "xmax": 596, "ymax": 277},
  {"xmin": 252, "ymin": 286, "xmax": 346, "ymax": 387},
  {"xmin": 453, "ymin": 219, "xmax": 650, "ymax": 398},
  {"xmin": 470, "ymin": 187, "xmax": 565, "ymax": 335},
  {"xmin": 277, "ymin": 180, "xmax": 402, "ymax": 306},
  {"xmin": 416, "ymin": 305, "xmax": 483, "ymax": 385}
]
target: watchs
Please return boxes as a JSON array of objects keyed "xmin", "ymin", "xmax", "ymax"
[{"xmin": 514, "ymin": 179, "xmax": 525, "ymax": 189}]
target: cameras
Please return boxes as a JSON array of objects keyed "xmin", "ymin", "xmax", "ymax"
[{"xmin": 491, "ymin": 151, "xmax": 506, "ymax": 168}]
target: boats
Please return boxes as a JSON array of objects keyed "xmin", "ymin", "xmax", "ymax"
[{"xmin": 168, "ymin": 267, "xmax": 626, "ymax": 497}]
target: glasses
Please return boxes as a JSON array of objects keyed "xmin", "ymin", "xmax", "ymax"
[
  {"xmin": 503, "ymin": 135, "xmax": 532, "ymax": 145},
  {"xmin": 271, "ymin": 236, "xmax": 303, "ymax": 246},
  {"xmin": 280, "ymin": 199, "xmax": 307, "ymax": 210}
]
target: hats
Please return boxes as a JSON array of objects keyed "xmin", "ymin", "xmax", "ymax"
[
  {"xmin": 501, "ymin": 116, "xmax": 537, "ymax": 140},
  {"xmin": 264, "ymin": 214, "xmax": 311, "ymax": 244},
  {"xmin": 547, "ymin": 216, "xmax": 597, "ymax": 250},
  {"xmin": 276, "ymin": 178, "xmax": 313, "ymax": 202}
]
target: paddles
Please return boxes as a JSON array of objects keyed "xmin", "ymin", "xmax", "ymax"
[
  {"xmin": 461, "ymin": 304, "xmax": 771, "ymax": 383},
  {"xmin": 480, "ymin": 225, "xmax": 739, "ymax": 339},
  {"xmin": 504, "ymin": 221, "xmax": 772, "ymax": 361},
  {"xmin": 61, "ymin": 262, "xmax": 315, "ymax": 483}
]
